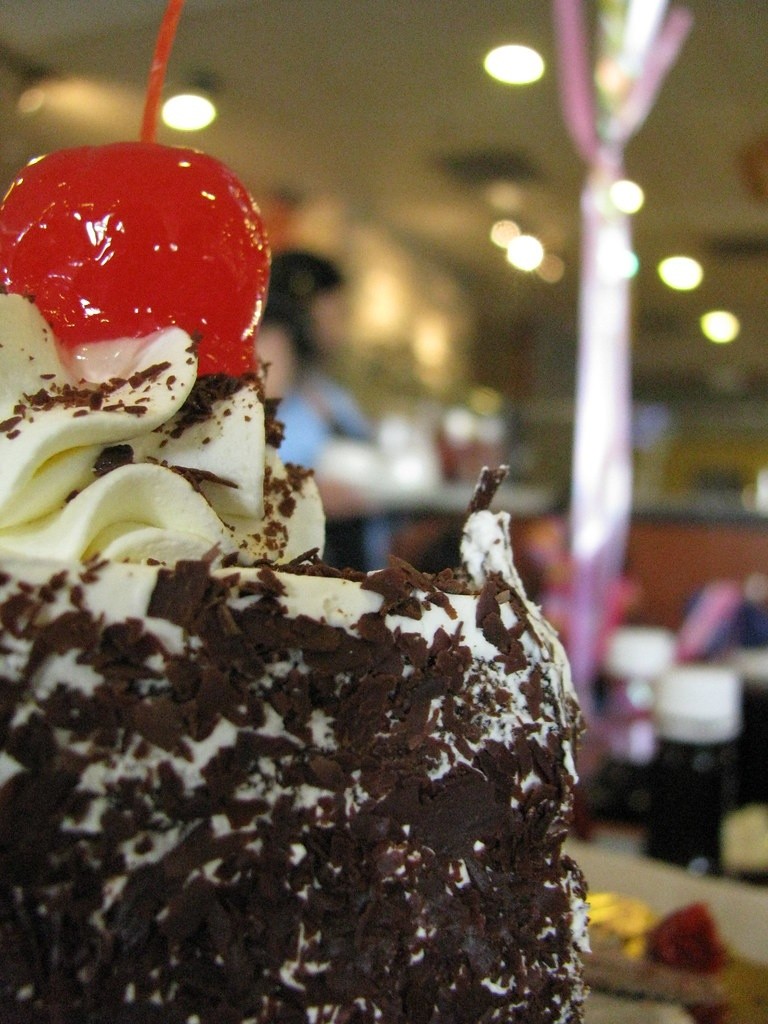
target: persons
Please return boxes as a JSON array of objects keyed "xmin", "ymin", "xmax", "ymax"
[{"xmin": 257, "ymin": 247, "xmax": 438, "ymax": 572}]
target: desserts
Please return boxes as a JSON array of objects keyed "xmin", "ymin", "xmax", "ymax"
[{"xmin": 0, "ymin": 0, "xmax": 589, "ymax": 1024}]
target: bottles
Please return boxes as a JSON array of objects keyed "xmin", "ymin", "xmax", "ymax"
[{"xmin": 630, "ymin": 665, "xmax": 747, "ymax": 871}]
